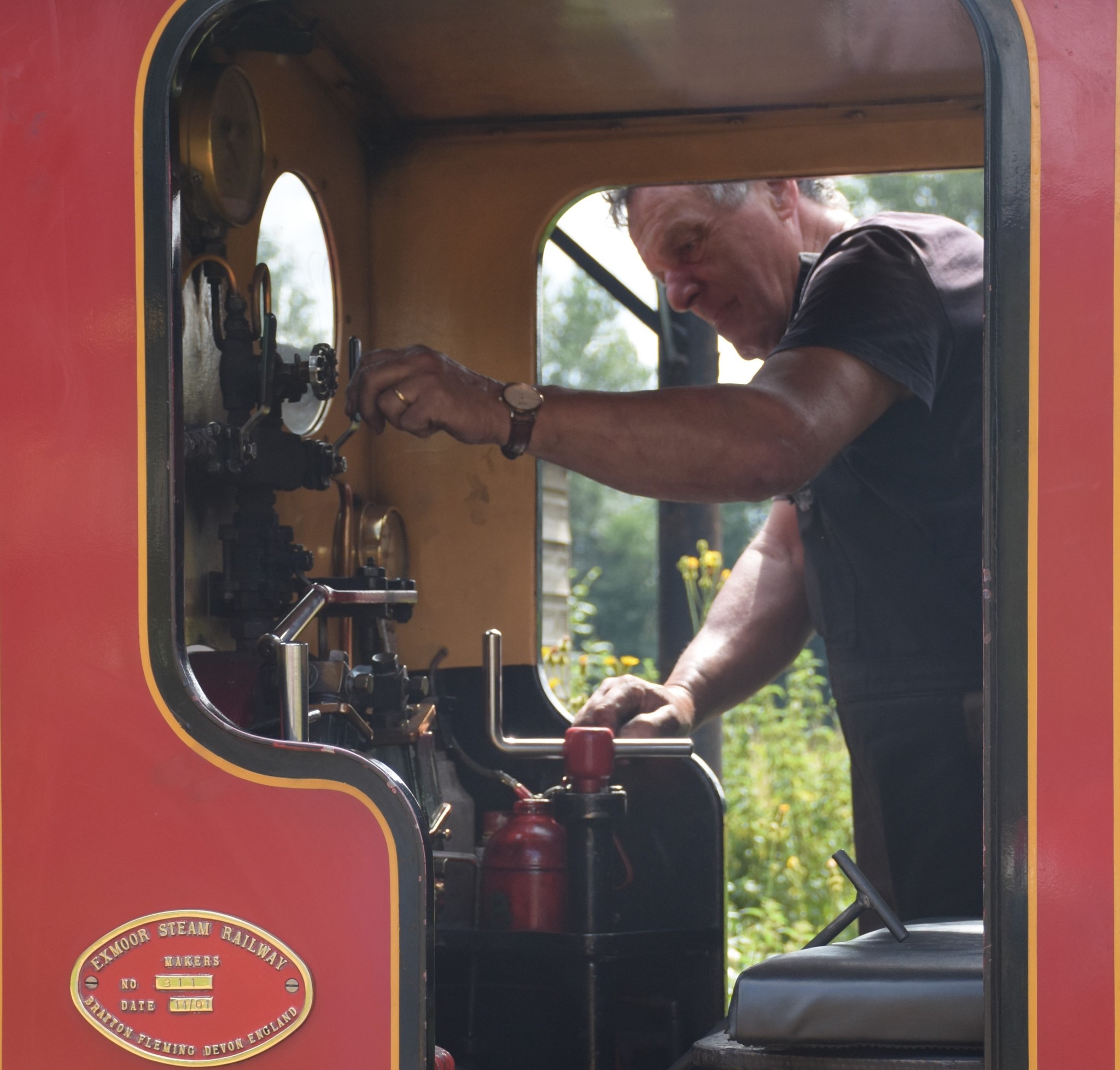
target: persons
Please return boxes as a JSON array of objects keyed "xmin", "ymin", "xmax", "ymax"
[{"xmin": 345, "ymin": 176, "xmax": 982, "ymax": 933}]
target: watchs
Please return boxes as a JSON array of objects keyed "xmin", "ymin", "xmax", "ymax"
[{"xmin": 498, "ymin": 380, "xmax": 546, "ymax": 462}]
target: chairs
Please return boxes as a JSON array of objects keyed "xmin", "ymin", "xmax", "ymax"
[{"xmin": 676, "ymin": 917, "xmax": 988, "ymax": 1070}]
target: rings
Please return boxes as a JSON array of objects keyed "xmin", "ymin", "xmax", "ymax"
[{"xmin": 390, "ymin": 385, "xmax": 412, "ymax": 408}]
